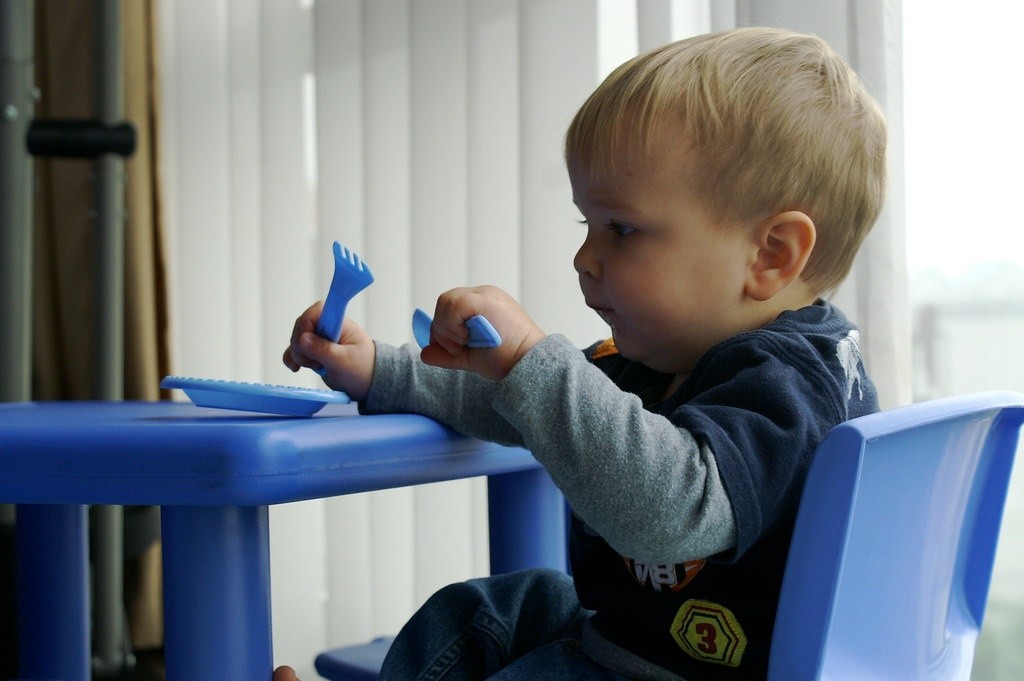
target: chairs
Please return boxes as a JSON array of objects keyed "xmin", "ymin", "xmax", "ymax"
[{"xmin": 768, "ymin": 389, "xmax": 1024, "ymax": 681}]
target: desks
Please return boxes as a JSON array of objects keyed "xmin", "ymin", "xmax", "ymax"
[{"xmin": 0, "ymin": 399, "xmax": 571, "ymax": 681}]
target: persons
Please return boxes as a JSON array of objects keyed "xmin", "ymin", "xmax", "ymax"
[{"xmin": 273, "ymin": 27, "xmax": 888, "ymax": 680}]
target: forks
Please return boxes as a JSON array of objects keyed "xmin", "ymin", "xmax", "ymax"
[{"xmin": 313, "ymin": 241, "xmax": 374, "ymax": 375}]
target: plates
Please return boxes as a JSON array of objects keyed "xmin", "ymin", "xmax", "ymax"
[{"xmin": 158, "ymin": 375, "xmax": 350, "ymax": 419}]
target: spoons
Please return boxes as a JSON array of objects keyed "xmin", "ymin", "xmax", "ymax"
[{"xmin": 412, "ymin": 308, "xmax": 502, "ymax": 349}]
